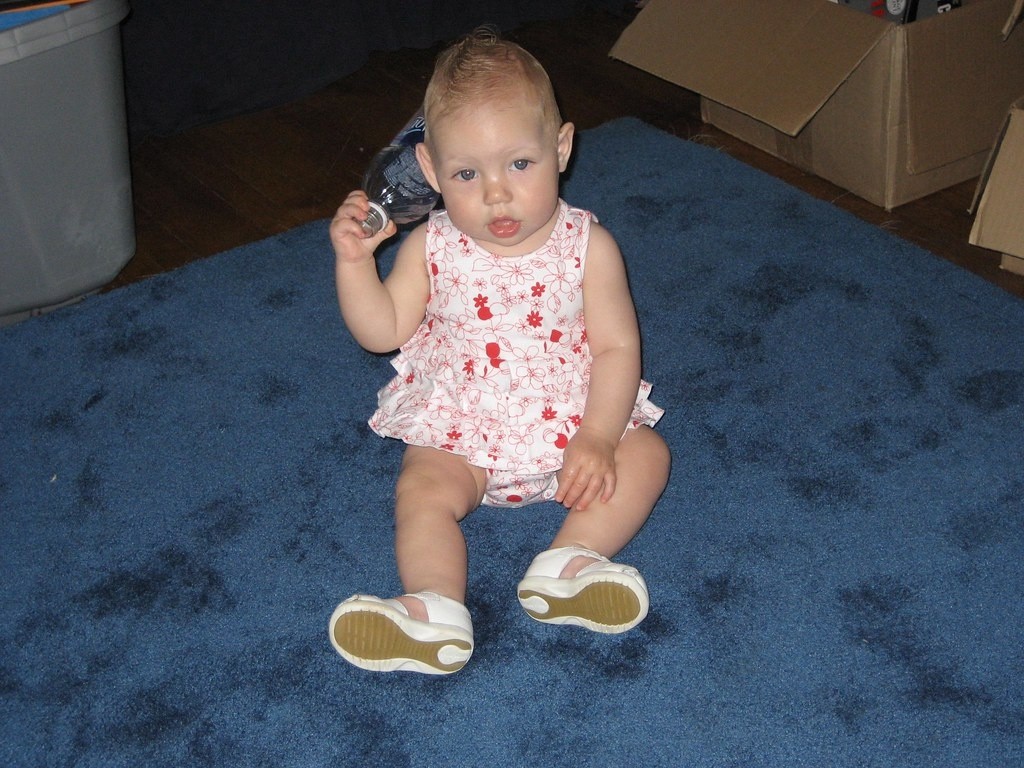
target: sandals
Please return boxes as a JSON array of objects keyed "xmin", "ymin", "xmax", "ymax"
[
  {"xmin": 517, "ymin": 546, "xmax": 649, "ymax": 634},
  {"xmin": 328, "ymin": 591, "xmax": 474, "ymax": 675}
]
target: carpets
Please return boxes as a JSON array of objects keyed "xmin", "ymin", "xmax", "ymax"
[{"xmin": 0, "ymin": 113, "xmax": 1024, "ymax": 768}]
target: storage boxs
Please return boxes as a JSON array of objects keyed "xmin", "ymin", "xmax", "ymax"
[
  {"xmin": 967, "ymin": 96, "xmax": 1024, "ymax": 276},
  {"xmin": 608, "ymin": 0, "xmax": 1024, "ymax": 211}
]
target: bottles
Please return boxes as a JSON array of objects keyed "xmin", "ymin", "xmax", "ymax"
[{"xmin": 354, "ymin": 100, "xmax": 442, "ymax": 237}]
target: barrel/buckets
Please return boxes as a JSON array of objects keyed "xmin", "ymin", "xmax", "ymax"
[{"xmin": 0, "ymin": 0, "xmax": 137, "ymax": 316}]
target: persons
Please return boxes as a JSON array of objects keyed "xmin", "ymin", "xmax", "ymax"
[{"xmin": 327, "ymin": 25, "xmax": 670, "ymax": 674}]
